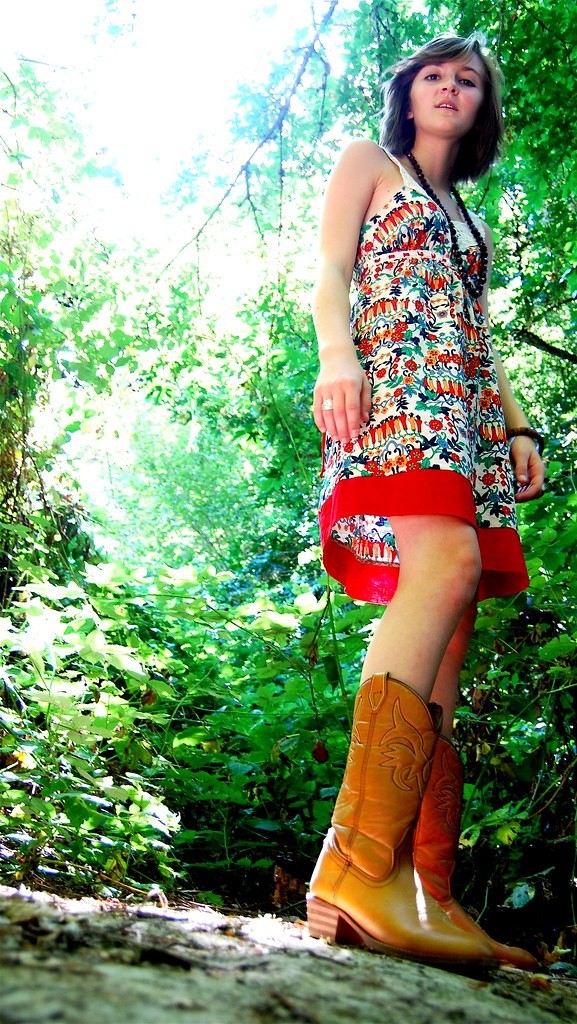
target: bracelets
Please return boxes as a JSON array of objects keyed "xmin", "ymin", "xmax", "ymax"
[{"xmin": 506, "ymin": 427, "xmax": 544, "ymax": 454}]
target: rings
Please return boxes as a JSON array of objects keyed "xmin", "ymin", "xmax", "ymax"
[{"xmin": 321, "ymin": 400, "xmax": 332, "ymax": 411}]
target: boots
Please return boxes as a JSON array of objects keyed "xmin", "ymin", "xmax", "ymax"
[
  {"xmin": 306, "ymin": 671, "xmax": 500, "ymax": 969},
  {"xmin": 413, "ymin": 732, "xmax": 539, "ymax": 970}
]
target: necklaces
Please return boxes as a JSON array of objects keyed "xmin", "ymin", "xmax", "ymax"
[{"xmin": 408, "ymin": 153, "xmax": 488, "ymax": 298}]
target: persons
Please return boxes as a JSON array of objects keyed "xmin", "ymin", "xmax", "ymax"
[{"xmin": 305, "ymin": 34, "xmax": 545, "ymax": 975}]
